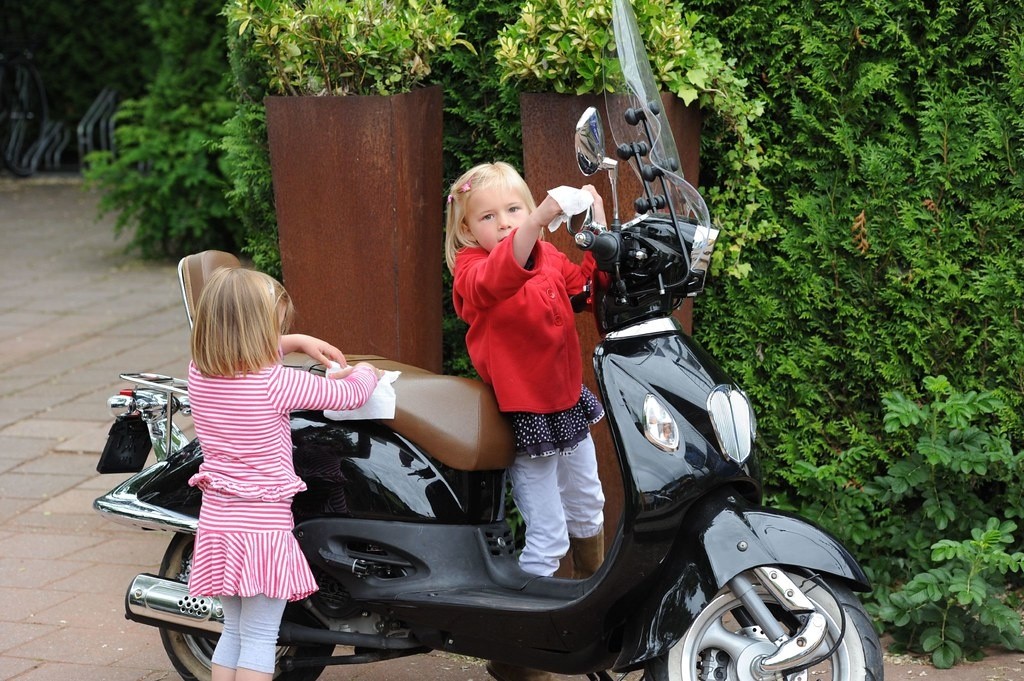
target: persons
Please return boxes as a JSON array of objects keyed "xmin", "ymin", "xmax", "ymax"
[
  {"xmin": 445, "ymin": 160, "xmax": 606, "ymax": 580},
  {"xmin": 187, "ymin": 265, "xmax": 386, "ymax": 681}
]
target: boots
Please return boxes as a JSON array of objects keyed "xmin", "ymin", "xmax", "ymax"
[
  {"xmin": 571, "ymin": 534, "xmax": 604, "ymax": 580},
  {"xmin": 487, "ymin": 659, "xmax": 550, "ymax": 681}
]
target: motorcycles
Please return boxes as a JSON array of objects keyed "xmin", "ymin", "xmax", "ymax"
[{"xmin": 89, "ymin": 0, "xmax": 890, "ymax": 681}]
